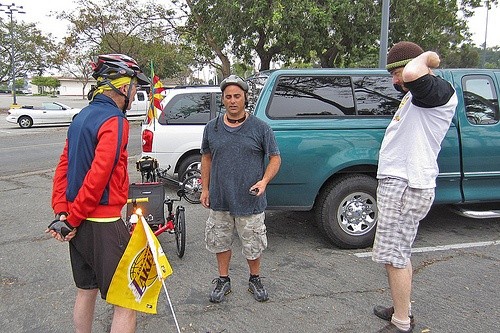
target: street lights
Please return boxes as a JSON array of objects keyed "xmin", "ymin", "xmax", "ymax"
[{"xmin": 0, "ymin": 3, "xmax": 27, "ymax": 109}]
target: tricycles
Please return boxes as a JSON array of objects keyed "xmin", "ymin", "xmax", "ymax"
[
  {"xmin": 124, "ymin": 158, "xmax": 187, "ymax": 258},
  {"xmin": 135, "ymin": 156, "xmax": 204, "ymax": 204}
]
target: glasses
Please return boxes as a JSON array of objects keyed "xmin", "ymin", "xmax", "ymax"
[{"xmin": 225, "ymin": 112, "xmax": 247, "ymax": 124}]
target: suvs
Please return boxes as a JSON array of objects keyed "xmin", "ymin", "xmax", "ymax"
[
  {"xmin": 140, "ymin": 83, "xmax": 264, "ymax": 191},
  {"xmin": 251, "ymin": 67, "xmax": 500, "ymax": 251}
]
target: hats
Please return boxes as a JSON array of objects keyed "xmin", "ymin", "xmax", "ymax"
[{"xmin": 385, "ymin": 41, "xmax": 425, "ymax": 71}]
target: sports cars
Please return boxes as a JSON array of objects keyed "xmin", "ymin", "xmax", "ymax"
[{"xmin": 6, "ymin": 101, "xmax": 83, "ymax": 129}]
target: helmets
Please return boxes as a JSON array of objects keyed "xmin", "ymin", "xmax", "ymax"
[
  {"xmin": 88, "ymin": 53, "xmax": 151, "ymax": 85},
  {"xmin": 219, "ymin": 74, "xmax": 249, "ymax": 92}
]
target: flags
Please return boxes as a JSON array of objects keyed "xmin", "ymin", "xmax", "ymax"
[
  {"xmin": 105, "ymin": 215, "xmax": 172, "ymax": 313},
  {"xmin": 145, "ymin": 60, "xmax": 166, "ymax": 124}
]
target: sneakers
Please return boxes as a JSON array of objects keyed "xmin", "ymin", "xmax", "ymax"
[
  {"xmin": 208, "ymin": 275, "xmax": 232, "ymax": 304},
  {"xmin": 373, "ymin": 304, "xmax": 414, "ymax": 325},
  {"xmin": 376, "ymin": 322, "xmax": 413, "ymax": 333},
  {"xmin": 248, "ymin": 274, "xmax": 269, "ymax": 302}
]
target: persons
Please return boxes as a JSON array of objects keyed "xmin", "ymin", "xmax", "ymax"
[
  {"xmin": 373, "ymin": 40, "xmax": 458, "ymax": 332},
  {"xmin": 45, "ymin": 54, "xmax": 150, "ymax": 333},
  {"xmin": 199, "ymin": 76, "xmax": 281, "ymax": 302}
]
